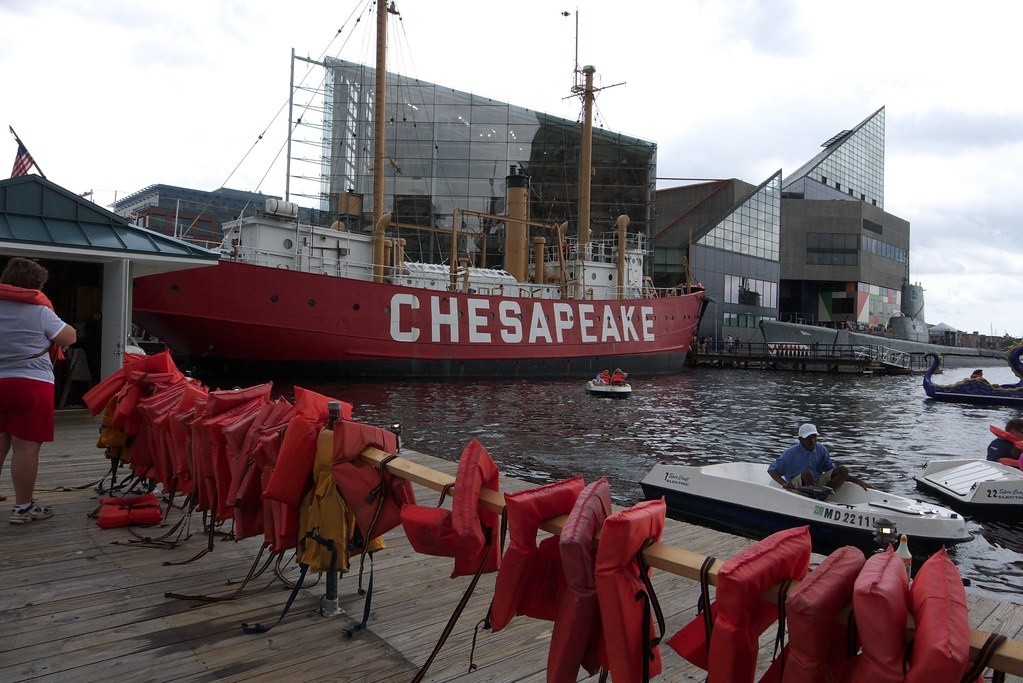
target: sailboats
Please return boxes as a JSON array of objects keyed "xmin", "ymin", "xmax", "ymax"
[{"xmin": 123, "ymin": 0, "xmax": 711, "ymax": 378}]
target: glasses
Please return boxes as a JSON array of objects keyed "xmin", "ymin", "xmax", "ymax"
[{"xmin": 615, "ymin": 371, "xmax": 621, "ymax": 374}]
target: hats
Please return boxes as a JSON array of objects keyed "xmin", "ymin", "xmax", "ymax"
[{"xmin": 797, "ymin": 423, "xmax": 821, "ymax": 438}]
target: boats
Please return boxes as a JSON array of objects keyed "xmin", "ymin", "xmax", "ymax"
[
  {"xmin": 584, "ymin": 379, "xmax": 633, "ymax": 397},
  {"xmin": 638, "ymin": 461, "xmax": 975, "ymax": 564},
  {"xmin": 921, "ymin": 340, "xmax": 1023, "ymax": 403},
  {"xmin": 911, "ymin": 457, "xmax": 1023, "ymax": 518}
]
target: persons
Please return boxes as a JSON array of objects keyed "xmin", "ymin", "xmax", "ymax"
[
  {"xmin": 596, "ymin": 368, "xmax": 611, "ymax": 384},
  {"xmin": 610, "ymin": 368, "xmax": 628, "ymax": 385},
  {"xmin": 0, "ymin": 256, "xmax": 79, "ymax": 523},
  {"xmin": 986, "ymin": 418, "xmax": 1023, "ymax": 464},
  {"xmin": 688, "ymin": 330, "xmax": 745, "ymax": 355},
  {"xmin": 767, "ymin": 423, "xmax": 875, "ymax": 501},
  {"xmin": 840, "ymin": 317, "xmax": 894, "ymax": 338},
  {"xmin": 971, "ymin": 370, "xmax": 980, "ymax": 379},
  {"xmin": 975, "ymin": 370, "xmax": 999, "ymax": 389}
]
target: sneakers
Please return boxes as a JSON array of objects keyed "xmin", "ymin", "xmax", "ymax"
[{"xmin": 8, "ymin": 500, "xmax": 54, "ymax": 525}]
